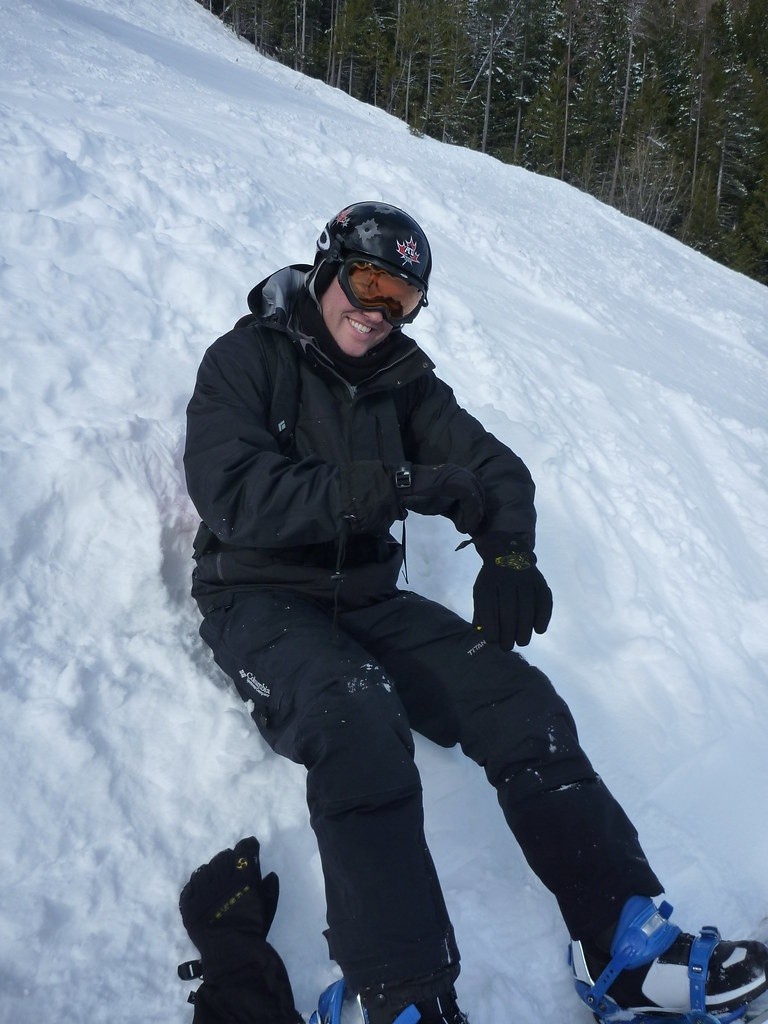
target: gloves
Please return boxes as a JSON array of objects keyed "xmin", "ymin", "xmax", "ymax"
[
  {"xmin": 473, "ymin": 539, "xmax": 553, "ymax": 653},
  {"xmin": 390, "ymin": 460, "xmax": 484, "ymax": 535},
  {"xmin": 180, "ymin": 837, "xmax": 305, "ymax": 1024}
]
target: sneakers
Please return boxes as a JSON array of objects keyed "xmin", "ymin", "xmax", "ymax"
[
  {"xmin": 307, "ymin": 978, "xmax": 470, "ymax": 1024},
  {"xmin": 569, "ymin": 895, "xmax": 767, "ymax": 1021}
]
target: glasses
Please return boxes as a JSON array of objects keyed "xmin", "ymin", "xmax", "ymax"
[{"xmin": 338, "ymin": 254, "xmax": 426, "ymax": 327}]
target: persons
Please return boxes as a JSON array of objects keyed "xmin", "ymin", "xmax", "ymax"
[{"xmin": 182, "ymin": 200, "xmax": 767, "ymax": 1024}]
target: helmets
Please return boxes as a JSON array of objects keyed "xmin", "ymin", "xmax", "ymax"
[{"xmin": 317, "ymin": 201, "xmax": 433, "ymax": 295}]
